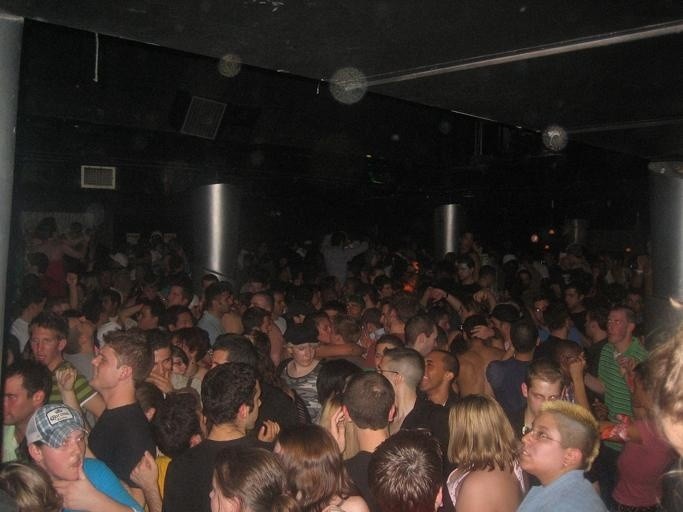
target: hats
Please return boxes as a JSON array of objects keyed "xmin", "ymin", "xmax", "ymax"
[
  {"xmin": 25, "ymin": 404, "xmax": 89, "ymax": 448},
  {"xmin": 491, "ymin": 305, "xmax": 520, "ymax": 323},
  {"xmin": 283, "ymin": 324, "xmax": 319, "ymax": 345}
]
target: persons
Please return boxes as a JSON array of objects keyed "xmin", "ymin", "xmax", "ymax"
[
  {"xmin": 455, "ymin": 232, "xmax": 656, "ymax": 319},
  {"xmin": 210, "ymin": 446, "xmax": 301, "ymax": 512},
  {"xmin": 61, "ymin": 316, "xmax": 96, "ymax": 382},
  {"xmin": 644, "ymin": 323, "xmax": 683, "ymax": 456},
  {"xmin": 485, "ymin": 321, "xmax": 542, "ymax": 437},
  {"xmin": 371, "ymin": 428, "xmax": 445, "ymax": 511},
  {"xmin": 306, "ymin": 231, "xmax": 455, "ymax": 359},
  {"xmin": 145, "ymin": 330, "xmax": 201, "ymax": 388},
  {"xmin": 152, "ymin": 390, "xmax": 210, "ymax": 499},
  {"xmin": 88, "ymin": 329, "xmax": 157, "ymax": 510},
  {"xmin": 512, "ymin": 351, "xmax": 590, "ymax": 436},
  {"xmin": 517, "ymin": 402, "xmax": 609, "ymax": 511},
  {"xmin": 275, "ymin": 423, "xmax": 369, "ymax": 512},
  {"xmin": 378, "ymin": 346, "xmax": 449, "ymax": 456},
  {"xmin": 213, "ymin": 332, "xmax": 296, "ymax": 430},
  {"xmin": 171, "ymin": 327, "xmax": 210, "ymax": 380},
  {"xmin": 534, "ymin": 301, "xmax": 582, "ymax": 369},
  {"xmin": 331, "ymin": 372, "xmax": 396, "ymax": 505},
  {"xmin": 165, "ymin": 363, "xmax": 280, "ymax": 512},
  {"xmin": 597, "ymin": 359, "xmax": 679, "ymax": 511},
  {"xmin": 27, "ymin": 404, "xmax": 146, "ymax": 512},
  {"xmin": 582, "ymin": 307, "xmax": 608, "ymax": 372},
  {"xmin": 276, "ymin": 322, "xmax": 323, "ymax": 422},
  {"xmin": 456, "ymin": 316, "xmax": 511, "ymax": 399},
  {"xmin": 420, "ymin": 348, "xmax": 464, "ymax": 408},
  {"xmin": 445, "ymin": 395, "xmax": 525, "ymax": 512},
  {"xmin": 30, "ymin": 311, "xmax": 108, "ymax": 419},
  {"xmin": 1, "ymin": 358, "xmax": 53, "ymax": 461},
  {"xmin": 8, "ymin": 217, "xmax": 305, "ymax": 332},
  {"xmin": 584, "ymin": 306, "xmax": 652, "ymax": 505}
]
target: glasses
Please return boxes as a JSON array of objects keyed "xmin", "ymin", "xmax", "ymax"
[
  {"xmin": 522, "ymin": 426, "xmax": 560, "ymax": 444},
  {"xmin": 376, "ymin": 367, "xmax": 394, "ymax": 373}
]
